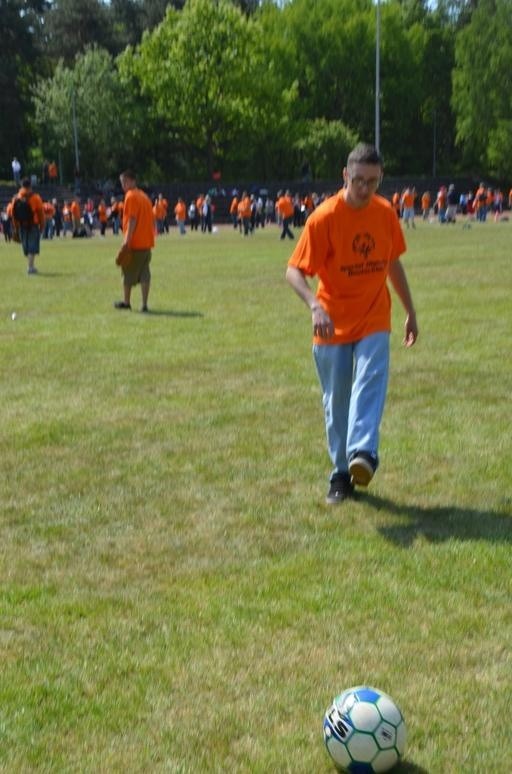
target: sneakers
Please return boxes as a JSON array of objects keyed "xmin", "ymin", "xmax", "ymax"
[
  {"xmin": 351, "ymin": 452, "xmax": 378, "ymax": 489},
  {"xmin": 28, "ymin": 269, "xmax": 38, "ymax": 273},
  {"xmin": 141, "ymin": 307, "xmax": 149, "ymax": 313},
  {"xmin": 115, "ymin": 301, "xmax": 131, "ymax": 311},
  {"xmin": 326, "ymin": 476, "xmax": 351, "ymax": 506}
]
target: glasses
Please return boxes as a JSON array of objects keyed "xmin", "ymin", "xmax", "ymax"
[{"xmin": 345, "ymin": 172, "xmax": 382, "ymax": 187}]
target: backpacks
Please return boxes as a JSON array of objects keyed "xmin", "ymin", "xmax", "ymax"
[{"xmin": 14, "ymin": 195, "xmax": 32, "ymax": 238}]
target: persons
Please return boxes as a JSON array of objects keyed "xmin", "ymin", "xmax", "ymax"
[
  {"xmin": 7, "ymin": 179, "xmax": 44, "ymax": 274},
  {"xmin": 286, "ymin": 143, "xmax": 419, "ymax": 504},
  {"xmin": 392, "ymin": 183, "xmax": 512, "ymax": 228},
  {"xmin": 48, "ymin": 161, "xmax": 59, "ymax": 187},
  {"xmin": 231, "ymin": 186, "xmax": 338, "ymax": 240},
  {"xmin": 29, "ymin": 166, "xmax": 39, "ymax": 191},
  {"xmin": 11, "ymin": 157, "xmax": 20, "ymax": 181},
  {"xmin": 0, "ymin": 196, "xmax": 126, "ymax": 242},
  {"xmin": 114, "ymin": 172, "xmax": 154, "ymax": 311},
  {"xmin": 153, "ymin": 184, "xmax": 226, "ymax": 233}
]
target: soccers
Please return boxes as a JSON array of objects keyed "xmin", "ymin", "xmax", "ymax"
[{"xmin": 322, "ymin": 685, "xmax": 407, "ymax": 774}]
